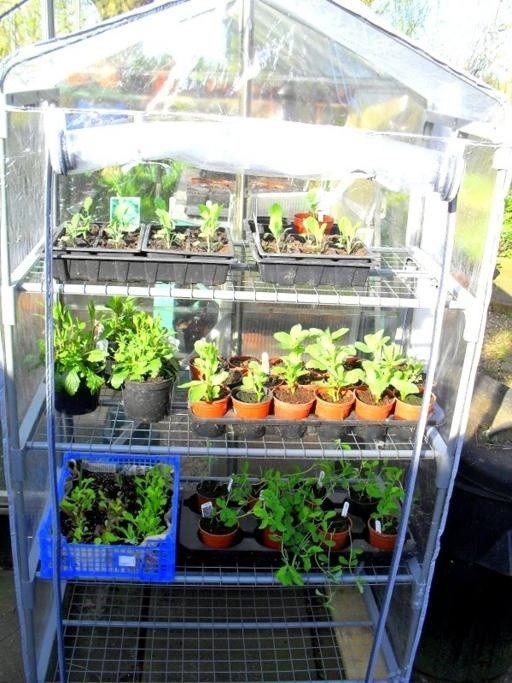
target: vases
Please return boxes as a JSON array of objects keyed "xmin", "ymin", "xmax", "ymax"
[{"xmin": 351, "ymin": 457, "xmax": 407, "ymax": 550}]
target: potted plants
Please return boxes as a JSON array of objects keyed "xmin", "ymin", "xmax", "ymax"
[
  {"xmin": 142, "ymin": 223, "xmax": 234, "ymax": 286},
  {"xmin": 21, "ymin": 300, "xmax": 109, "ymax": 416},
  {"xmin": 98, "ymin": 295, "xmax": 144, "ymax": 392},
  {"xmin": 269, "ymin": 324, "xmax": 310, "ymax": 370},
  {"xmin": 353, "ymin": 353, "xmax": 396, "ymax": 439},
  {"xmin": 231, "ymin": 361, "xmax": 273, "ymax": 436},
  {"xmin": 390, "ymin": 359, "xmax": 437, "ymax": 439},
  {"xmin": 189, "ymin": 341, "xmax": 227, "ymax": 381},
  {"xmin": 356, "ymin": 330, "xmax": 413, "ymax": 371},
  {"xmin": 38, "ymin": 449, "xmax": 179, "ymax": 583},
  {"xmin": 177, "ymin": 366, "xmax": 231, "ymax": 438},
  {"xmin": 293, "ymin": 191, "xmax": 334, "ymax": 234},
  {"xmin": 50, "ymin": 221, "xmax": 145, "ymax": 282},
  {"xmin": 271, "ymin": 353, "xmax": 315, "ymax": 439},
  {"xmin": 110, "ymin": 311, "xmax": 179, "ymax": 423},
  {"xmin": 306, "ymin": 343, "xmax": 365, "ymax": 438},
  {"xmin": 254, "ymin": 232, "xmax": 374, "ymax": 288},
  {"xmin": 178, "ymin": 439, "xmax": 364, "ymax": 610},
  {"xmin": 307, "ymin": 328, "xmax": 350, "ymax": 381}
]
